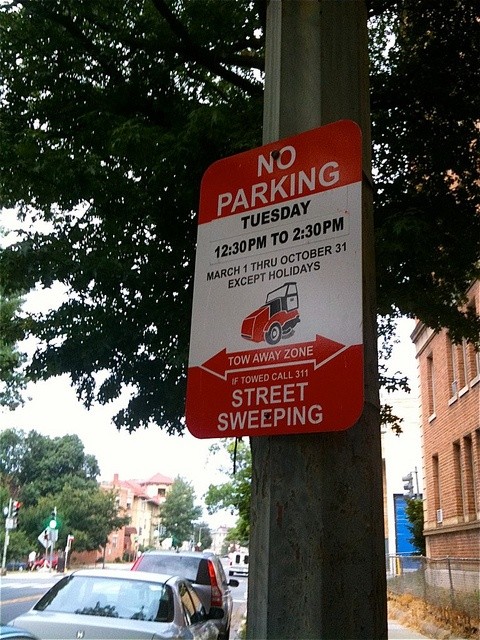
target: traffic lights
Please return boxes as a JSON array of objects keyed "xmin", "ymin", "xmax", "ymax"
[
  {"xmin": 49, "ymin": 511, "xmax": 57, "ymax": 528},
  {"xmin": 402, "ymin": 473, "xmax": 414, "ymax": 498},
  {"xmin": 12, "ymin": 501, "xmax": 23, "ymax": 518}
]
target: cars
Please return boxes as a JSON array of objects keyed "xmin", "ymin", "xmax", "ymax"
[
  {"xmin": 228, "ymin": 551, "xmax": 249, "ymax": 576},
  {"xmin": 130, "ymin": 552, "xmax": 239, "ymax": 640},
  {"xmin": 31, "ymin": 553, "xmax": 59, "ymax": 571},
  {"xmin": 8, "ymin": 570, "xmax": 224, "ymax": 640},
  {"xmin": 6, "ymin": 558, "xmax": 28, "ymax": 571}
]
